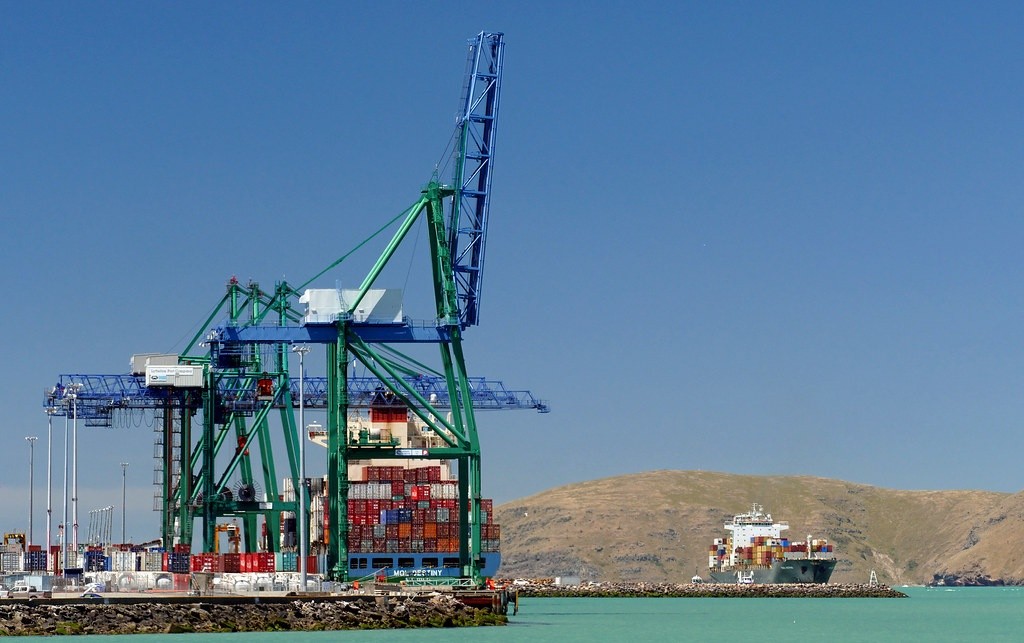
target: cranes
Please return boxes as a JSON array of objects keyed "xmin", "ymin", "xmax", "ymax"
[{"xmin": 44, "ymin": 352, "xmax": 551, "ymax": 555}]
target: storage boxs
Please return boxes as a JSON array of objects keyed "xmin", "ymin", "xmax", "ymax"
[
  {"xmin": 708, "ymin": 536, "xmax": 833, "ymax": 569},
  {"xmin": 0, "ymin": 543, "xmax": 284, "ymax": 575},
  {"xmin": 130, "ymin": 353, "xmax": 203, "ymax": 388},
  {"xmin": 348, "ymin": 466, "xmax": 501, "ymax": 553},
  {"xmin": 298, "ymin": 288, "xmax": 403, "ymax": 327},
  {"xmin": 283, "ymin": 477, "xmax": 329, "ymax": 574}
]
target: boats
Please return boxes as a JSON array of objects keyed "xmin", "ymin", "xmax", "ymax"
[
  {"xmin": 691, "ymin": 574, "xmax": 704, "ymax": 583},
  {"xmin": 736, "ymin": 570, "xmax": 755, "ymax": 584}
]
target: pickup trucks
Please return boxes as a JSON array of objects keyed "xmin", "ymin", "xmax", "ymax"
[{"xmin": 6, "ymin": 584, "xmax": 53, "ymax": 599}]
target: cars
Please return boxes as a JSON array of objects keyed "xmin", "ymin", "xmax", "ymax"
[{"xmin": 513, "ymin": 579, "xmax": 530, "ymax": 585}]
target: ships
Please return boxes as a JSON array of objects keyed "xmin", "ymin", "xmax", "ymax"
[
  {"xmin": 707, "ymin": 503, "xmax": 838, "ymax": 585},
  {"xmin": 259, "ymin": 391, "xmax": 505, "ymax": 583}
]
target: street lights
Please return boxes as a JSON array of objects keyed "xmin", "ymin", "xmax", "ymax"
[{"xmin": 25, "ymin": 382, "xmax": 84, "ymax": 551}]
